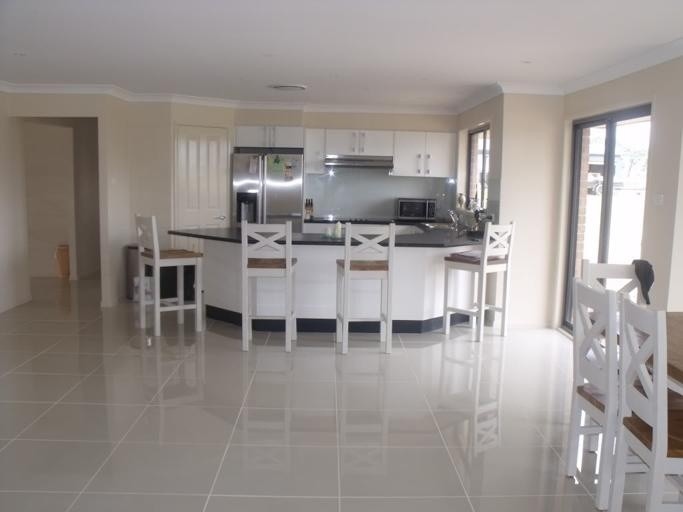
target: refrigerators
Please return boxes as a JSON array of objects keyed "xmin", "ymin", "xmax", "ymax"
[{"xmin": 230, "ymin": 146, "xmax": 304, "ymax": 233}]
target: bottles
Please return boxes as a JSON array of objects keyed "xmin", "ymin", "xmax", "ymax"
[{"xmin": 335, "ymin": 221, "xmax": 342, "ymax": 240}]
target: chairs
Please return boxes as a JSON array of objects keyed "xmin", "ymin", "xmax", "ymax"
[
  {"xmin": 443, "ymin": 220, "xmax": 516, "ymax": 342},
  {"xmin": 242, "ymin": 218, "xmax": 397, "ymax": 355},
  {"xmin": 136, "ymin": 212, "xmax": 206, "ymax": 337},
  {"xmin": 566, "ymin": 258, "xmax": 682, "ymax": 511}
]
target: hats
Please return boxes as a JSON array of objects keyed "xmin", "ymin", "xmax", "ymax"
[{"xmin": 632, "ymin": 259, "xmax": 654, "ymax": 304}]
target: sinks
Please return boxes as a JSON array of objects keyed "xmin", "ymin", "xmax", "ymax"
[{"xmin": 421, "ymin": 222, "xmax": 454, "ymax": 229}]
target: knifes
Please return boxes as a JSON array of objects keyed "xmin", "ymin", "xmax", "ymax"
[{"xmin": 306, "ymin": 198, "xmax": 314, "ymax": 207}]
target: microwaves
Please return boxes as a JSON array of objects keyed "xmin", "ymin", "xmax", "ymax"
[{"xmin": 395, "ymin": 197, "xmax": 437, "ymax": 223}]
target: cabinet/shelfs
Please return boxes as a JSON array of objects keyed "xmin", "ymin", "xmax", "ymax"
[
  {"xmin": 325, "ymin": 129, "xmax": 393, "ymax": 156},
  {"xmin": 304, "ymin": 128, "xmax": 325, "ymax": 175},
  {"xmin": 388, "ymin": 130, "xmax": 456, "ymax": 179},
  {"xmin": 236, "ymin": 126, "xmax": 304, "ymax": 148}
]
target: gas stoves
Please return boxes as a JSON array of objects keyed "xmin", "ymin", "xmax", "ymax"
[{"xmin": 307, "ymin": 216, "xmax": 391, "ymax": 224}]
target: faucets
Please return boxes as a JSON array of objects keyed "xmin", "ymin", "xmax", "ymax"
[{"xmin": 447, "ymin": 209, "xmax": 459, "ymax": 225}]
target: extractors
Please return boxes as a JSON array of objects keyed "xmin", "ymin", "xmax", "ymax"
[{"xmin": 323, "ymin": 154, "xmax": 393, "ymax": 172}]
